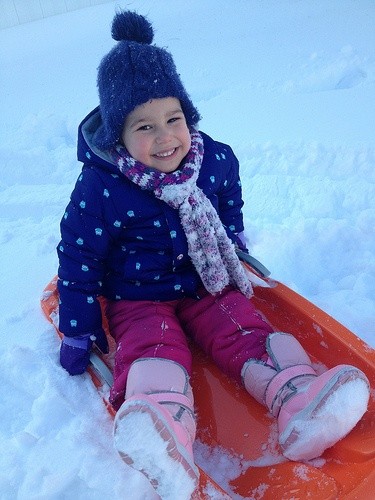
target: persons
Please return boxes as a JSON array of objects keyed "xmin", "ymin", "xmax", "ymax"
[{"xmin": 56, "ymin": 10, "xmax": 370, "ymax": 499}]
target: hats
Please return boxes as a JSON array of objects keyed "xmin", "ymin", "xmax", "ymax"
[{"xmin": 96, "ymin": 4, "xmax": 203, "ymax": 142}]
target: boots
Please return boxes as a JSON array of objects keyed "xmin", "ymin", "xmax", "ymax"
[
  {"xmin": 113, "ymin": 357, "xmax": 200, "ymax": 500},
  {"xmin": 241, "ymin": 332, "xmax": 371, "ymax": 461}
]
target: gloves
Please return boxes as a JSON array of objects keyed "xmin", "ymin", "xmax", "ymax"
[{"xmin": 60, "ymin": 327, "xmax": 109, "ymax": 376}]
